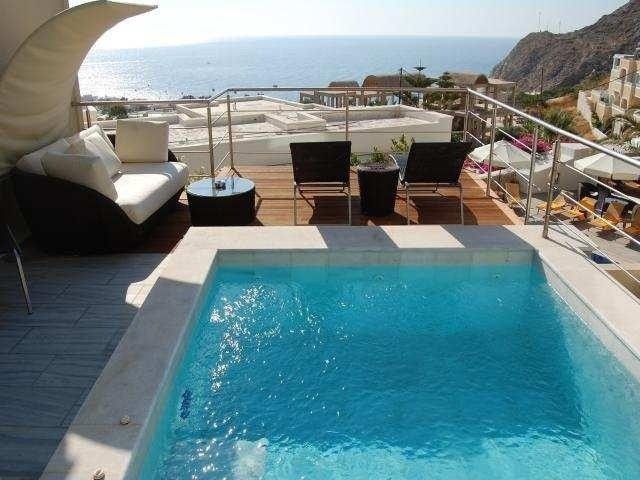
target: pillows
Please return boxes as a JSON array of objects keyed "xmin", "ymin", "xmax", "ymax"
[{"xmin": 16, "ymin": 119, "xmax": 170, "ymax": 203}]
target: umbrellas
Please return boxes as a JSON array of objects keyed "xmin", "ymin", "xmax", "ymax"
[
  {"xmin": 471, "ymin": 136, "xmax": 532, "ymax": 191},
  {"xmin": 575, "ymin": 148, "xmax": 640, "ymax": 217}
]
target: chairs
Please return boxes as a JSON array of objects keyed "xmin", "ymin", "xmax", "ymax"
[
  {"xmin": 504, "ymin": 183, "xmax": 524, "ymax": 212},
  {"xmin": 536, "ymin": 190, "xmax": 639, "ymax": 248},
  {"xmin": 388, "ymin": 141, "xmax": 472, "ymax": 225},
  {"xmin": 290, "ymin": 141, "xmax": 352, "ymax": 226}
]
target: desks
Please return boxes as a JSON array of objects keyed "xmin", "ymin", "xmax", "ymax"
[{"xmin": 357, "ymin": 163, "xmax": 400, "ymax": 218}]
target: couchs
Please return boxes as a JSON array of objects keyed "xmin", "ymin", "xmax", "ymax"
[{"xmin": 8, "ymin": 133, "xmax": 184, "ymax": 253}]
